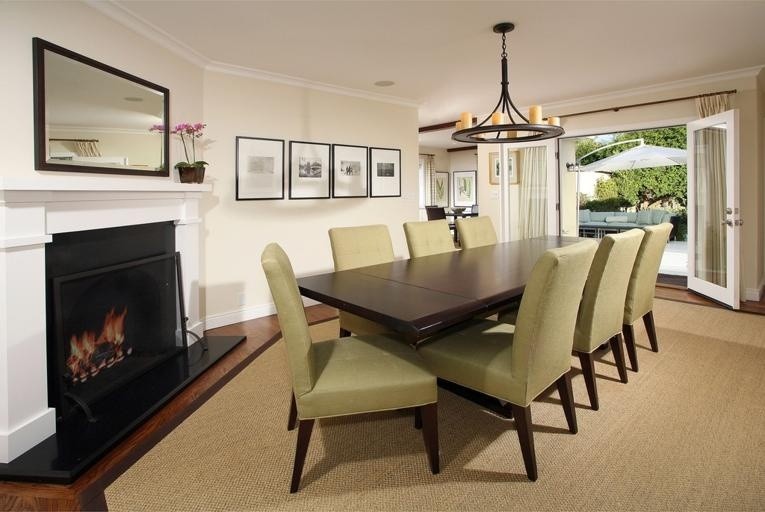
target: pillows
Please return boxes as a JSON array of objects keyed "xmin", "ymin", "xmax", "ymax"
[{"xmin": 579, "ymin": 209, "xmax": 671, "ymax": 224}]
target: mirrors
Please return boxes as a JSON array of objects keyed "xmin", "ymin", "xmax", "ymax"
[{"xmin": 33, "ymin": 37, "xmax": 170, "ymax": 177}]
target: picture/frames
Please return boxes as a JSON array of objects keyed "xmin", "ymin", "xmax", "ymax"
[
  {"xmin": 435, "ymin": 172, "xmax": 449, "ymax": 208},
  {"xmin": 289, "ymin": 140, "xmax": 330, "ymax": 199},
  {"xmin": 369, "ymin": 147, "xmax": 401, "ymax": 198},
  {"xmin": 332, "ymin": 144, "xmax": 368, "ymax": 198},
  {"xmin": 489, "ymin": 151, "xmax": 520, "ymax": 185},
  {"xmin": 453, "ymin": 171, "xmax": 477, "ymax": 207},
  {"xmin": 236, "ymin": 136, "xmax": 284, "ymax": 200}
]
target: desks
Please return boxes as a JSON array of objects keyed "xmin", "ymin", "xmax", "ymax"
[
  {"xmin": 293, "ymin": 233, "xmax": 622, "ymax": 432},
  {"xmin": 445, "ymin": 213, "xmax": 478, "ymax": 247}
]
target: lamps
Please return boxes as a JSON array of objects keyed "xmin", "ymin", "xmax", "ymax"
[{"xmin": 452, "ymin": 23, "xmax": 565, "ymax": 143}]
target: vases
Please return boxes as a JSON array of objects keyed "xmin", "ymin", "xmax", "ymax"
[
  {"xmin": 192, "ymin": 167, "xmax": 205, "ymax": 184},
  {"xmin": 178, "ymin": 167, "xmax": 196, "ymax": 183},
  {"xmin": 156, "ymin": 168, "xmax": 164, "ymax": 171}
]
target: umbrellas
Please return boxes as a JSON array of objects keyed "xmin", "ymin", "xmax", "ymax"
[{"xmin": 577, "ymin": 138, "xmax": 688, "ymax": 237}]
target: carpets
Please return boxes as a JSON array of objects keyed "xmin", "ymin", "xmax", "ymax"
[{"xmin": 78, "ymin": 297, "xmax": 765, "ymax": 512}]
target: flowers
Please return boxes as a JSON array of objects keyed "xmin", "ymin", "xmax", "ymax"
[
  {"xmin": 186, "ymin": 122, "xmax": 209, "ymax": 168},
  {"xmin": 149, "ymin": 125, "xmax": 165, "ymax": 167},
  {"xmin": 169, "ymin": 122, "xmax": 192, "ymax": 169}
]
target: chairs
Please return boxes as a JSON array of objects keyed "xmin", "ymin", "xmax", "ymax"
[
  {"xmin": 428, "ymin": 207, "xmax": 455, "ymax": 242},
  {"xmin": 620, "ymin": 223, "xmax": 673, "ymax": 370},
  {"xmin": 402, "ymin": 218, "xmax": 460, "ymax": 257},
  {"xmin": 327, "ymin": 221, "xmax": 424, "ymax": 340},
  {"xmin": 260, "ymin": 241, "xmax": 444, "ymax": 491},
  {"xmin": 425, "ymin": 205, "xmax": 438, "ymax": 212},
  {"xmin": 497, "ymin": 219, "xmax": 643, "ymax": 414},
  {"xmin": 453, "ymin": 217, "xmax": 499, "ymax": 250},
  {"xmin": 412, "ymin": 237, "xmax": 600, "ymax": 481}
]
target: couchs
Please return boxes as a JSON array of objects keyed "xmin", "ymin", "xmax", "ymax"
[{"xmin": 579, "ymin": 209, "xmax": 672, "ymax": 237}]
